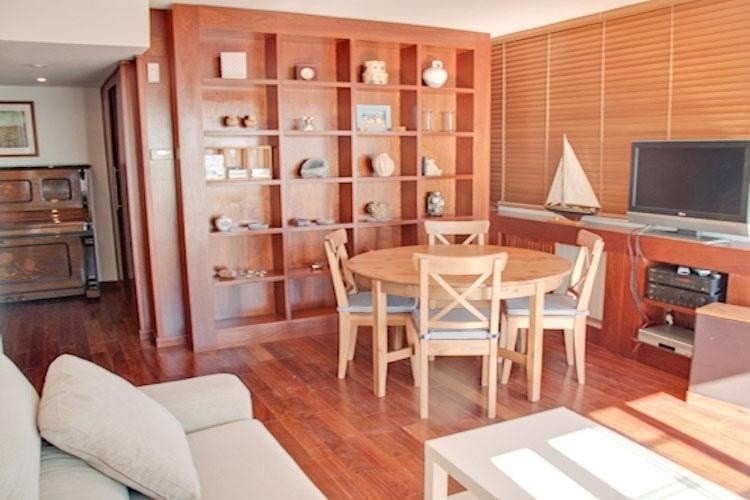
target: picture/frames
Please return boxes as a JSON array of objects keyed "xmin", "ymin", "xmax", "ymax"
[{"xmin": 1, "ymin": 101, "xmax": 38, "ymax": 156}]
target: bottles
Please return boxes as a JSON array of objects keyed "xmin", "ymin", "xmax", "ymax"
[
  {"xmin": 426, "ymin": 191, "xmax": 445, "ymax": 216},
  {"xmin": 423, "ymin": 59, "xmax": 448, "ymax": 89}
]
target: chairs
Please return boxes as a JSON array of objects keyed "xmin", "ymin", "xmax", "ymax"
[
  {"xmin": 496, "ymin": 229, "xmax": 605, "ymax": 384},
  {"xmin": 324, "ymin": 229, "xmax": 435, "ymax": 387},
  {"xmin": 423, "ymin": 216, "xmax": 490, "ymax": 363},
  {"xmin": 406, "ymin": 251, "xmax": 509, "ymax": 421}
]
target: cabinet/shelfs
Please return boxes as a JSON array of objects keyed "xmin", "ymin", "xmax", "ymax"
[
  {"xmin": 174, "ymin": 3, "xmax": 493, "ymax": 354},
  {"xmin": 489, "ymin": 210, "xmax": 749, "ymax": 381},
  {"xmin": 0, "ymin": 163, "xmax": 103, "ymax": 305}
]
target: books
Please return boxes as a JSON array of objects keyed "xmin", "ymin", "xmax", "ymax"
[{"xmin": 200, "ymin": 143, "xmax": 275, "ymax": 181}]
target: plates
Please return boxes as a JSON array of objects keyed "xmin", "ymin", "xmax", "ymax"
[
  {"xmin": 317, "ymin": 219, "xmax": 334, "ymax": 225},
  {"xmin": 248, "ymin": 223, "xmax": 270, "ymax": 230}
]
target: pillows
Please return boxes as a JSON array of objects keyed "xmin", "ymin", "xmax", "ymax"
[{"xmin": 36, "ymin": 355, "xmax": 201, "ymax": 499}]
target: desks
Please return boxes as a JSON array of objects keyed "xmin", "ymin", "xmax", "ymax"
[{"xmin": 347, "ymin": 243, "xmax": 572, "ymax": 399}]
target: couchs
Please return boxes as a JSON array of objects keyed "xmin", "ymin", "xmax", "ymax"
[{"xmin": 1, "ymin": 353, "xmax": 325, "ymax": 500}]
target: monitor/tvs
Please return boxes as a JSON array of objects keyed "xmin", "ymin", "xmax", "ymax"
[{"xmin": 627, "ymin": 140, "xmax": 750, "ymax": 246}]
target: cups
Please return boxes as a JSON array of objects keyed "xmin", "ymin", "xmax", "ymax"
[
  {"xmin": 440, "ymin": 112, "xmax": 453, "ymax": 131},
  {"xmin": 422, "ymin": 110, "xmax": 434, "ymax": 132}
]
